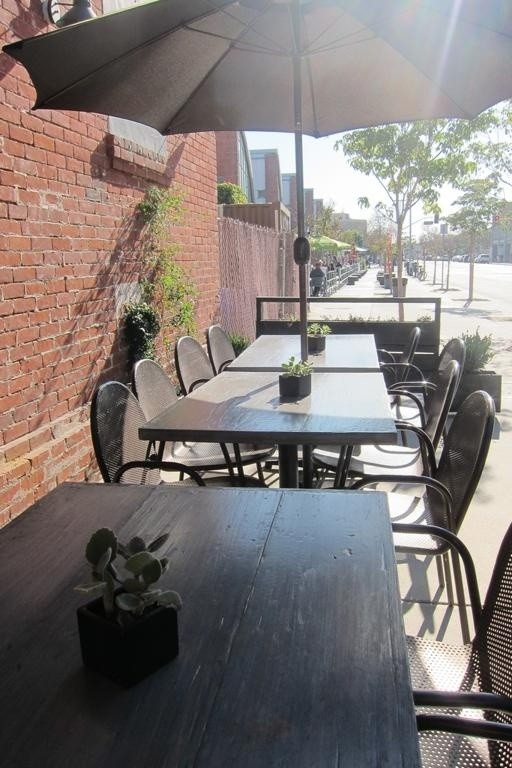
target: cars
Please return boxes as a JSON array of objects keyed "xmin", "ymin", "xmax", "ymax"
[{"xmin": 414, "ymin": 253, "xmax": 491, "ymax": 264}]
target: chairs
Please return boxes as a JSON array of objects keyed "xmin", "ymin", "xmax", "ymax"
[
  {"xmin": 132, "ymin": 356, "xmax": 279, "ymax": 485},
  {"xmin": 310, "ymin": 390, "xmax": 473, "ymax": 646},
  {"xmin": 377, "ymin": 337, "xmax": 466, "ymax": 452},
  {"xmin": 376, "ymin": 326, "xmax": 424, "ymax": 402},
  {"xmin": 84, "ymin": 379, "xmax": 268, "ymax": 489},
  {"xmin": 404, "ymin": 520, "xmax": 510, "ymax": 766},
  {"xmin": 173, "ymin": 334, "xmax": 216, "ymax": 397},
  {"xmin": 206, "ymin": 324, "xmax": 239, "ymax": 376}
]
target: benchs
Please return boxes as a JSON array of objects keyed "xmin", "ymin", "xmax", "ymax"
[{"xmin": 347, "ymin": 269, "xmax": 367, "ymax": 285}]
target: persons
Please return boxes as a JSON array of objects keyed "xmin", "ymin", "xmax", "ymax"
[{"xmin": 310, "ymin": 262, "xmax": 325, "ymax": 297}]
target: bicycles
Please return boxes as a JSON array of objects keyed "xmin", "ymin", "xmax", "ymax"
[{"xmin": 403, "ymin": 257, "xmax": 427, "ymax": 282}]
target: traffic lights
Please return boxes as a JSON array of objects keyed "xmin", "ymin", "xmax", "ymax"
[
  {"xmin": 434, "ymin": 214, "xmax": 439, "ymax": 224},
  {"xmin": 423, "ymin": 220, "xmax": 433, "ymax": 226}
]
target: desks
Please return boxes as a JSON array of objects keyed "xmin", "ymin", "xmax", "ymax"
[
  {"xmin": 138, "ymin": 369, "xmax": 398, "ymax": 490},
  {"xmin": 0, "ymin": 478, "xmax": 425, "ymax": 766},
  {"xmin": 224, "ymin": 333, "xmax": 380, "ymax": 489}
]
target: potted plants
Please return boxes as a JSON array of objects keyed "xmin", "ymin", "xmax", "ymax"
[
  {"xmin": 279, "ymin": 356, "xmax": 315, "ymax": 399},
  {"xmin": 74, "ymin": 525, "xmax": 183, "ymax": 680},
  {"xmin": 307, "ymin": 324, "xmax": 331, "ymax": 353}
]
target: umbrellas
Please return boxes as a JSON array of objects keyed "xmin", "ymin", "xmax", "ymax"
[
  {"xmin": 1, "ymin": 0, "xmax": 512, "ymax": 490},
  {"xmin": 312, "ymin": 234, "xmax": 368, "ymax": 266}
]
